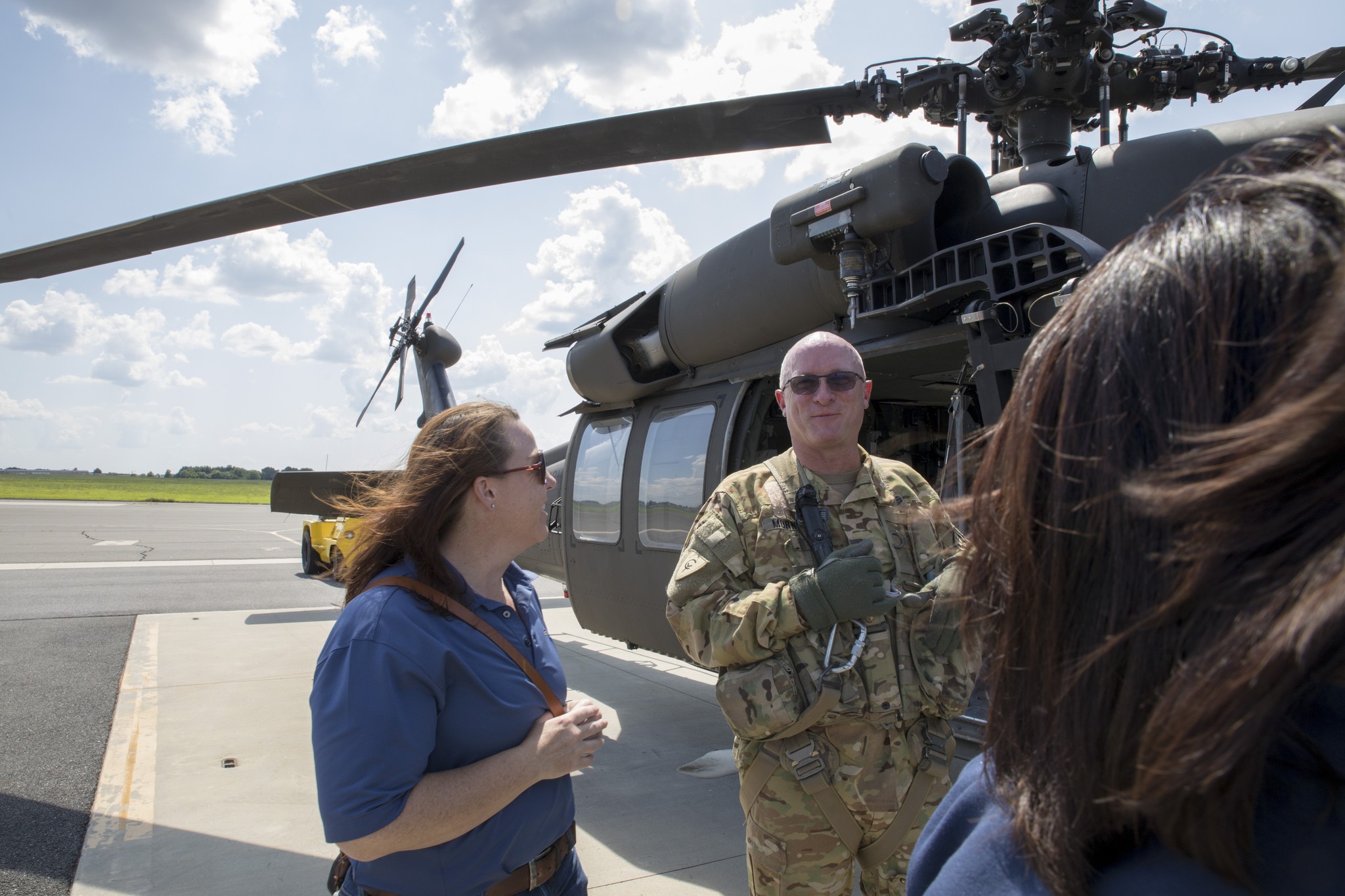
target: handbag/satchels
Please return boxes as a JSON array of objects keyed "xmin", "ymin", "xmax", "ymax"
[{"xmin": 326, "ymin": 851, "xmax": 352, "ymax": 896}]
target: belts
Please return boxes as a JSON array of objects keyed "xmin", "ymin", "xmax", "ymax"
[{"xmin": 353, "ymin": 821, "xmax": 579, "ymax": 896}]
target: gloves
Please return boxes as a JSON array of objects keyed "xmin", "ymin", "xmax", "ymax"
[
  {"xmin": 789, "ymin": 539, "xmax": 900, "ymax": 632},
  {"xmin": 903, "ymin": 549, "xmax": 979, "ymax": 654}
]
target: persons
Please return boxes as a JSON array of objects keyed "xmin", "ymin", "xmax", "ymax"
[
  {"xmin": 667, "ymin": 328, "xmax": 990, "ymax": 896},
  {"xmin": 907, "ymin": 126, "xmax": 1344, "ymax": 896},
  {"xmin": 310, "ymin": 403, "xmax": 624, "ymax": 896}
]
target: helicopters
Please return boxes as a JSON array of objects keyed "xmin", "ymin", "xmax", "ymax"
[{"xmin": 1, "ymin": 1, "xmax": 1344, "ymax": 763}]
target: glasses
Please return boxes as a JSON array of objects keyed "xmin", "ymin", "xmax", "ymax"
[
  {"xmin": 484, "ymin": 450, "xmax": 550, "ymax": 485},
  {"xmin": 782, "ymin": 371, "xmax": 866, "ymax": 394}
]
